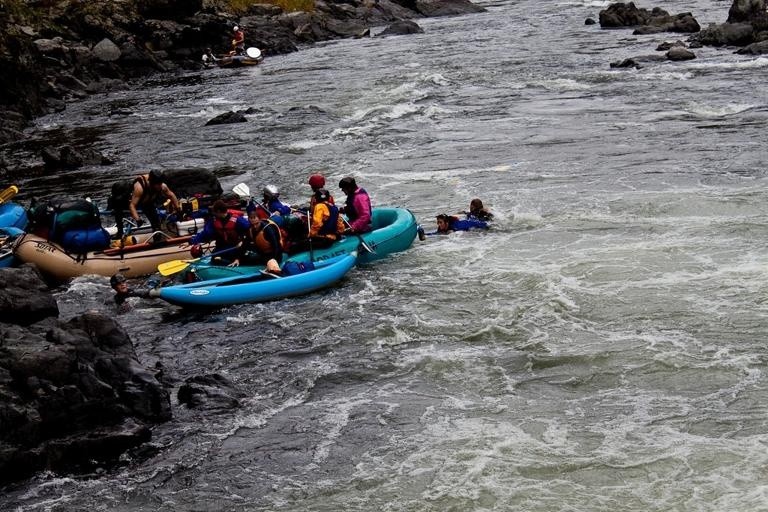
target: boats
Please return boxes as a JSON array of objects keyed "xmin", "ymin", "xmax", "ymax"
[
  {"xmin": 0, "ymin": 202, "xmax": 29, "ymax": 267},
  {"xmin": 186, "ymin": 206, "xmax": 416, "ymax": 282},
  {"xmin": 150, "ymin": 250, "xmax": 361, "ymax": 309},
  {"xmin": 216, "ymin": 54, "xmax": 264, "ymax": 67},
  {"xmin": 11, "ymin": 202, "xmax": 292, "ymax": 281}
]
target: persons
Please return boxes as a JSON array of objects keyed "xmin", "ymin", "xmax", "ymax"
[
  {"xmin": 232, "ymin": 26, "xmax": 245, "ymax": 56},
  {"xmin": 308, "ymin": 175, "xmax": 334, "ymax": 213},
  {"xmin": 201, "ymin": 48, "xmax": 222, "ymax": 70},
  {"xmin": 307, "ymin": 189, "xmax": 345, "ymax": 250},
  {"xmin": 424, "ymin": 214, "xmax": 489, "ymax": 235},
  {"xmin": 104, "ymin": 273, "xmax": 154, "ymax": 306},
  {"xmin": 186, "ymin": 245, "xmax": 203, "ymax": 283},
  {"xmin": 338, "ymin": 177, "xmax": 372, "ymax": 237},
  {"xmin": 111, "ymin": 169, "xmax": 180, "ymax": 243},
  {"xmin": 189, "ymin": 184, "xmax": 313, "ymax": 273},
  {"xmin": 467, "ymin": 199, "xmax": 494, "ymax": 222}
]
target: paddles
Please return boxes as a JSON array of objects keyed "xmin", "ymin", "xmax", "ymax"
[
  {"xmin": 232, "ymin": 183, "xmax": 271, "ymax": 216},
  {"xmin": 157, "ymin": 246, "xmax": 241, "ymax": 276},
  {"xmin": 341, "ymin": 216, "xmax": 378, "ymax": 255}
]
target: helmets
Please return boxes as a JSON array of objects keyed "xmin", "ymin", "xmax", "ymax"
[
  {"xmin": 110, "ymin": 274, "xmax": 127, "ymax": 288},
  {"xmin": 338, "ymin": 177, "xmax": 356, "ymax": 190},
  {"xmin": 190, "ymin": 245, "xmax": 203, "ymax": 257},
  {"xmin": 263, "ymin": 184, "xmax": 280, "ymax": 200},
  {"xmin": 308, "ymin": 174, "xmax": 325, "ymax": 190},
  {"xmin": 148, "ymin": 169, "xmax": 166, "ymax": 184}
]
